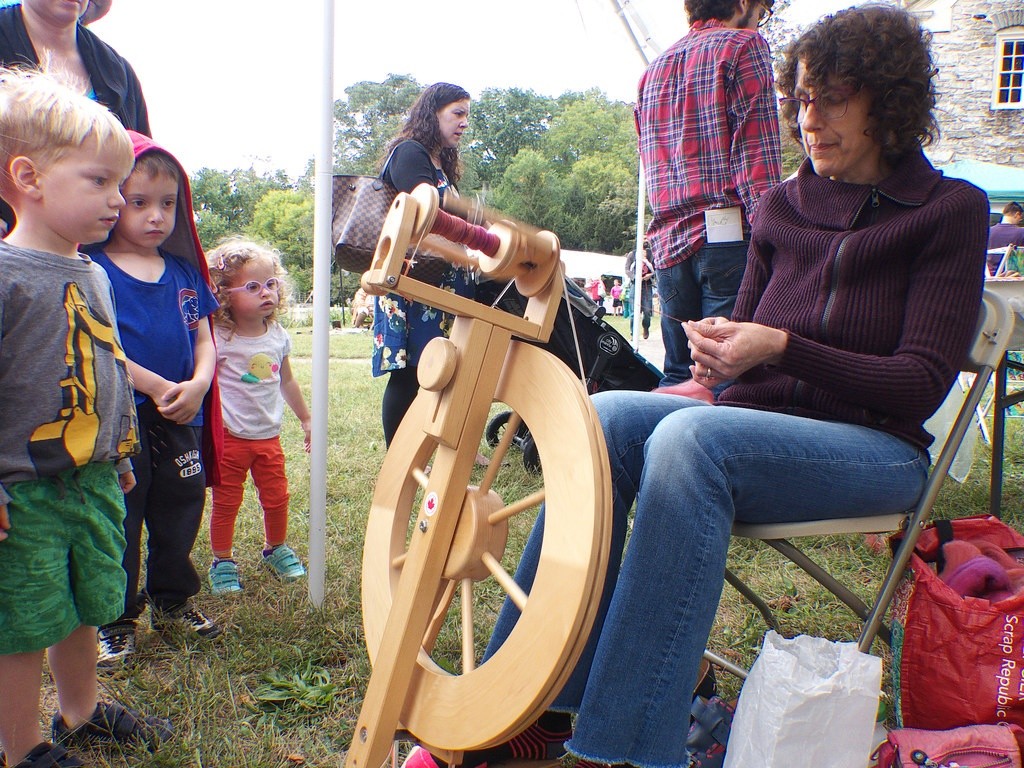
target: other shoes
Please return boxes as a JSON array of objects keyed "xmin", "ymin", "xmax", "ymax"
[
  {"xmin": 643, "ymin": 327, "xmax": 649, "ymax": 339},
  {"xmin": 631, "ymin": 336, "xmax": 633, "ymax": 340}
]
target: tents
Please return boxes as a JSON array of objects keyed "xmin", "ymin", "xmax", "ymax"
[{"xmin": 933, "ymin": 158, "xmax": 1024, "ymax": 201}]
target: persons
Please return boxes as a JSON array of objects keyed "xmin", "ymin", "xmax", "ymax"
[
  {"xmin": 0, "ymin": 67, "xmax": 174, "ymax": 768},
  {"xmin": 364, "ymin": 82, "xmax": 511, "ymax": 478},
  {"xmin": 402, "ymin": 5, "xmax": 989, "ymax": 768},
  {"xmin": 0, "ymin": 0, "xmax": 154, "ymax": 256},
  {"xmin": 634, "ymin": 1, "xmax": 783, "ymax": 388},
  {"xmin": 85, "ymin": 129, "xmax": 221, "ymax": 679},
  {"xmin": 202, "ymin": 236, "xmax": 311, "ymax": 597},
  {"xmin": 986, "ymin": 202, "xmax": 1024, "ymax": 276},
  {"xmin": 585, "ymin": 231, "xmax": 656, "ymax": 345}
]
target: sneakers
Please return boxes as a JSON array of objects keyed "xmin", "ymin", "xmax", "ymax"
[
  {"xmin": 51, "ymin": 701, "xmax": 173, "ymax": 752},
  {"xmin": 263, "ymin": 544, "xmax": 306, "ymax": 582},
  {"xmin": 208, "ymin": 558, "xmax": 241, "ymax": 596},
  {"xmin": 96, "ymin": 621, "xmax": 137, "ymax": 667},
  {"xmin": 152, "ymin": 599, "xmax": 220, "ymax": 640},
  {"xmin": 0, "ymin": 742, "xmax": 93, "ymax": 768}
]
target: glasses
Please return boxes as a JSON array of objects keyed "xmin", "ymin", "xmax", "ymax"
[
  {"xmin": 1019, "ymin": 212, "xmax": 1024, "ymax": 222},
  {"xmin": 225, "ymin": 277, "xmax": 280, "ymax": 296},
  {"xmin": 757, "ymin": 0, "xmax": 774, "ymax": 27},
  {"xmin": 778, "ymin": 85, "xmax": 869, "ymax": 124}
]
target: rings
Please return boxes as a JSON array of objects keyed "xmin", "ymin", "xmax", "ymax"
[{"xmin": 707, "ymin": 367, "xmax": 711, "ymax": 379}]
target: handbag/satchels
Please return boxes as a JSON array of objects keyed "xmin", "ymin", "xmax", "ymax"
[
  {"xmin": 333, "ymin": 140, "xmax": 436, "ymax": 273},
  {"xmin": 597, "ymin": 281, "xmax": 606, "ymax": 298},
  {"xmin": 618, "ymin": 289, "xmax": 626, "ymax": 301},
  {"xmin": 889, "ymin": 514, "xmax": 1024, "ymax": 766},
  {"xmin": 871, "ymin": 724, "xmax": 1022, "ymax": 768},
  {"xmin": 722, "ymin": 631, "xmax": 883, "ymax": 768}
]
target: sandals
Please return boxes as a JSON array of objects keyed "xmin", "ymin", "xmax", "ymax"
[{"xmin": 686, "ymin": 695, "xmax": 738, "ymax": 768}]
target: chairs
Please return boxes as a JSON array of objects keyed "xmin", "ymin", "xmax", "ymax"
[{"xmin": 703, "ymin": 287, "xmax": 1016, "ymax": 679}]
